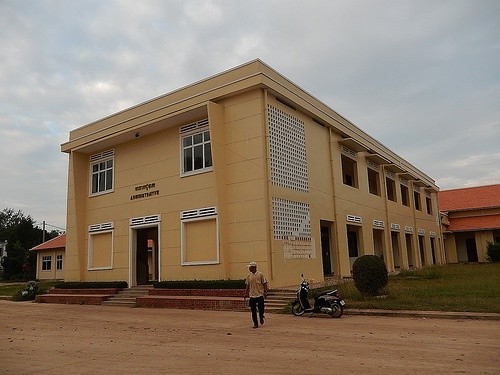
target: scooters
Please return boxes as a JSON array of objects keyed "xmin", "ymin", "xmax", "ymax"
[{"xmin": 291, "ymin": 273, "xmax": 346, "ymax": 318}]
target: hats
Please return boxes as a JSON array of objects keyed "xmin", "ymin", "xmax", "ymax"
[{"xmin": 246, "ymin": 262, "xmax": 258, "ymax": 267}]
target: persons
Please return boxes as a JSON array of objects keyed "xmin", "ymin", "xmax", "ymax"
[{"xmin": 244, "ymin": 262, "xmax": 268, "ymax": 328}]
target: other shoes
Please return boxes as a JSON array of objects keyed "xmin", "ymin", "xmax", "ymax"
[
  {"xmin": 259, "ymin": 317, "xmax": 264, "ymax": 325},
  {"xmin": 253, "ymin": 324, "xmax": 259, "ymax": 328}
]
